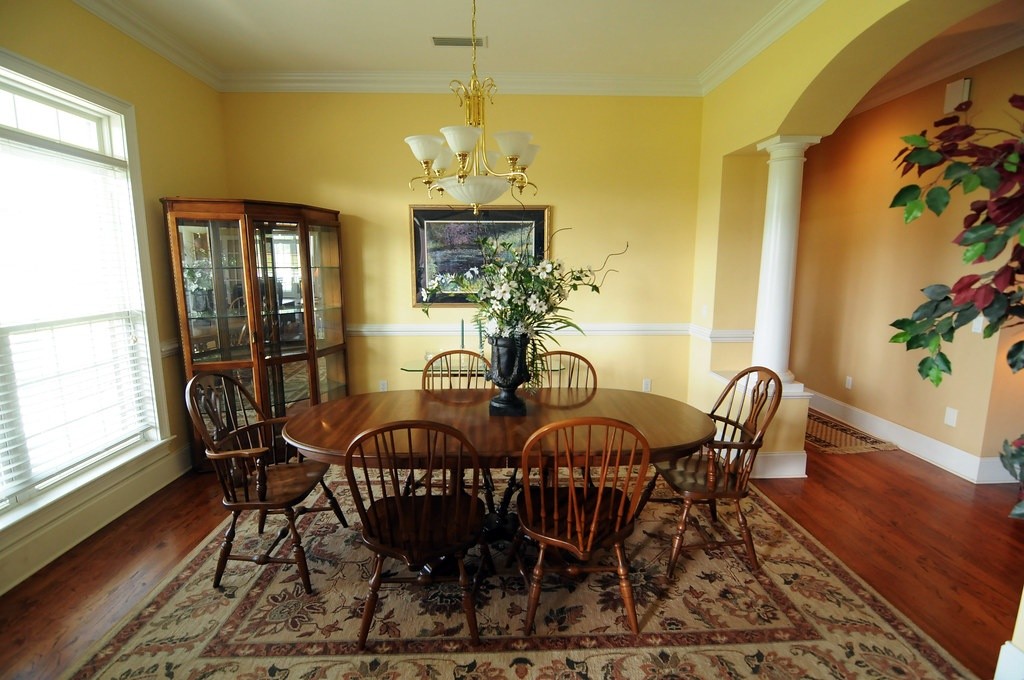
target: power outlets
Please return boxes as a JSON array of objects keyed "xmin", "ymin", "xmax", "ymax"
[{"xmin": 943, "ymin": 407, "xmax": 959, "ymax": 427}]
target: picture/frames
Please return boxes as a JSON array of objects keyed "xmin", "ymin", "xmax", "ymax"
[{"xmin": 409, "ymin": 204, "xmax": 552, "ymax": 310}]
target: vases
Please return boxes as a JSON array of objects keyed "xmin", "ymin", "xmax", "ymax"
[{"xmin": 485, "ymin": 336, "xmax": 531, "ymax": 417}]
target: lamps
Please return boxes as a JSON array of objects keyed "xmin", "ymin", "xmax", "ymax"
[
  {"xmin": 942, "ymin": 77, "xmax": 971, "ymax": 116},
  {"xmin": 404, "ymin": 0, "xmax": 540, "ymax": 217}
]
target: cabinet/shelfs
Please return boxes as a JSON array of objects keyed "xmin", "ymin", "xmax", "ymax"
[{"xmin": 158, "ymin": 196, "xmax": 349, "ymax": 474}]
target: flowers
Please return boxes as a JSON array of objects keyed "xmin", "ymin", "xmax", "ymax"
[{"xmin": 419, "ymin": 211, "xmax": 629, "ymax": 397}]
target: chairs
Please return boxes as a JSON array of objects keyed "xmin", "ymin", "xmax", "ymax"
[
  {"xmin": 185, "ymin": 371, "xmax": 350, "ymax": 595},
  {"xmin": 413, "ymin": 350, "xmax": 497, "ymax": 489},
  {"xmin": 344, "ymin": 418, "xmax": 500, "ymax": 649},
  {"xmin": 631, "ymin": 366, "xmax": 783, "ymax": 581},
  {"xmin": 520, "ymin": 350, "xmax": 597, "ymax": 488},
  {"xmin": 514, "ymin": 418, "xmax": 652, "ymax": 636}
]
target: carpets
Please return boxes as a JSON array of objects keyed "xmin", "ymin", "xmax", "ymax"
[
  {"xmin": 57, "ymin": 462, "xmax": 983, "ymax": 680},
  {"xmin": 805, "ymin": 412, "xmax": 898, "ymax": 454}
]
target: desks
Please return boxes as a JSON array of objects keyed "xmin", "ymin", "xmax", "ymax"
[
  {"xmin": 281, "ymin": 387, "xmax": 718, "ymax": 586},
  {"xmin": 401, "ymin": 360, "xmax": 565, "ymax": 389}
]
